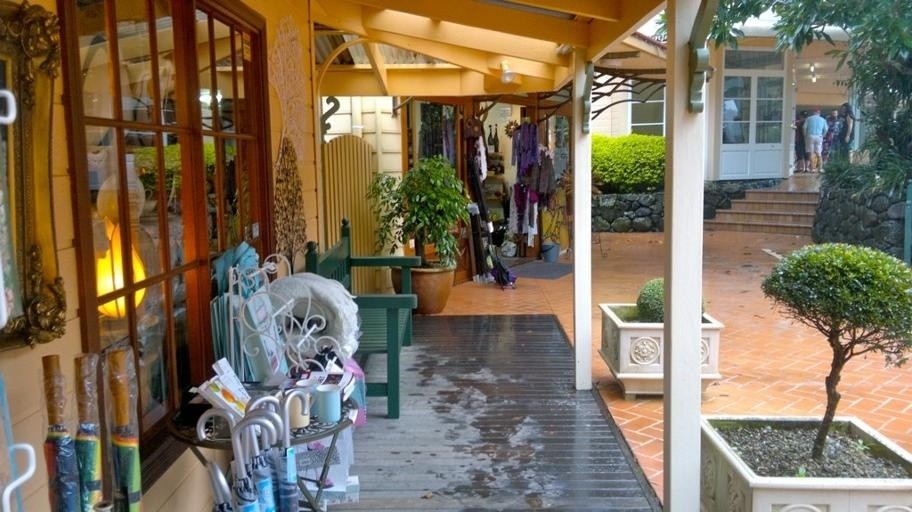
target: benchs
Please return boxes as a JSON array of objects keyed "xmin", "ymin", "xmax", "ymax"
[{"xmin": 303, "ymin": 217, "xmax": 422, "ymax": 420}]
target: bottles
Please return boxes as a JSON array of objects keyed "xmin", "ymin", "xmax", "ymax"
[
  {"xmin": 488, "ymin": 124, "xmax": 494, "ymax": 153},
  {"xmin": 493, "ymin": 123, "xmax": 500, "ymax": 152}
]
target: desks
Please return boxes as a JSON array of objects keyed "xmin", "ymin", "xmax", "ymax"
[{"xmin": 167, "ymin": 396, "xmax": 358, "ymax": 512}]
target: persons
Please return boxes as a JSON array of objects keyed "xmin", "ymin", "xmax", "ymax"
[
  {"xmin": 795, "ymin": 110, "xmax": 811, "ymax": 173},
  {"xmin": 829, "ymin": 108, "xmax": 845, "ymax": 159},
  {"xmin": 840, "ymin": 102, "xmax": 856, "ymax": 161},
  {"xmin": 801, "ymin": 106, "xmax": 829, "ymax": 174}
]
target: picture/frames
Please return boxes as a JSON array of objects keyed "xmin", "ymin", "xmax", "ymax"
[{"xmin": 0, "ymin": 1, "xmax": 68, "ymax": 352}]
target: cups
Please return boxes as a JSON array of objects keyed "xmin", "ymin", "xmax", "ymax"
[
  {"xmin": 296, "ymin": 379, "xmax": 318, "ymax": 417},
  {"xmin": 317, "ymin": 385, "xmax": 341, "ymax": 423},
  {"xmin": 285, "ymin": 390, "xmax": 310, "ymax": 429}
]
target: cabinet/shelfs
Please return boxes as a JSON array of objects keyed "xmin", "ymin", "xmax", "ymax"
[{"xmin": 481, "ymin": 153, "xmax": 505, "ymax": 225}]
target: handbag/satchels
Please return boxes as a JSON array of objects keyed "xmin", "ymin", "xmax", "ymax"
[
  {"xmin": 150, "ymin": 251, "xmax": 191, "ymax": 404},
  {"xmin": 208, "ymin": 285, "xmax": 290, "ymax": 383}
]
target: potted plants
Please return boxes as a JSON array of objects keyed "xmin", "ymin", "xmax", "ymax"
[
  {"xmin": 701, "ymin": 242, "xmax": 912, "ymax": 512},
  {"xmin": 364, "ymin": 154, "xmax": 473, "ymax": 312},
  {"xmin": 595, "ymin": 277, "xmax": 720, "ymax": 405}
]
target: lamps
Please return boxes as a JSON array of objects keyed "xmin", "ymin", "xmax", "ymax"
[
  {"xmin": 810, "ymin": 63, "xmax": 815, "ymax": 72},
  {"xmin": 812, "ymin": 73, "xmax": 817, "ymax": 83},
  {"xmin": 501, "ymin": 60, "xmax": 515, "ymax": 86}
]
